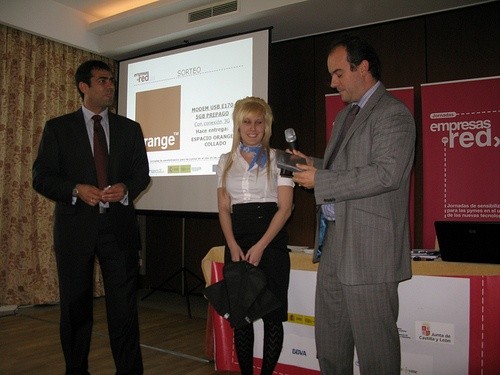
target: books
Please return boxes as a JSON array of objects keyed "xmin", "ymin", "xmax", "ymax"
[
  {"xmin": 410, "ymin": 247, "xmax": 440, "ymax": 260},
  {"xmin": 287, "ymin": 244, "xmax": 314, "ymax": 254}
]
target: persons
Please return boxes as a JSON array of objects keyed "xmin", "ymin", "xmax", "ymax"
[
  {"xmin": 217, "ymin": 96, "xmax": 295, "ymax": 375},
  {"xmin": 32, "ymin": 60, "xmax": 151, "ymax": 375},
  {"xmin": 285, "ymin": 44, "xmax": 414, "ymax": 375}
]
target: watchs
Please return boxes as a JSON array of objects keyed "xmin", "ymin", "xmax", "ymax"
[{"xmin": 72, "ymin": 188, "xmax": 78, "ymax": 196}]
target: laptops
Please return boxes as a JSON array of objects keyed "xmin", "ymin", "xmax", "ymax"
[{"xmin": 434, "ymin": 220, "xmax": 500, "ymax": 265}]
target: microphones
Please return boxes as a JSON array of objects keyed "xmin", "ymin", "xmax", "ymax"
[{"xmin": 285, "ymin": 128, "xmax": 296, "ymax": 152}]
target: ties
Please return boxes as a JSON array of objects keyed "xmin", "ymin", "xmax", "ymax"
[
  {"xmin": 91, "ymin": 115, "xmax": 109, "ymax": 190},
  {"xmin": 324, "ymin": 105, "xmax": 360, "ymax": 170}
]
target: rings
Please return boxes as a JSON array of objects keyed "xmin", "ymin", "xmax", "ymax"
[{"xmin": 299, "ymin": 184, "xmax": 301, "ymax": 186}]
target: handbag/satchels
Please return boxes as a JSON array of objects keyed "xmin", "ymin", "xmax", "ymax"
[{"xmin": 202, "ymin": 261, "xmax": 281, "ymax": 331}]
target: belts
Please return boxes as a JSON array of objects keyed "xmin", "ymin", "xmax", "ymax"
[{"xmin": 98, "ymin": 206, "xmax": 109, "ymax": 214}]
